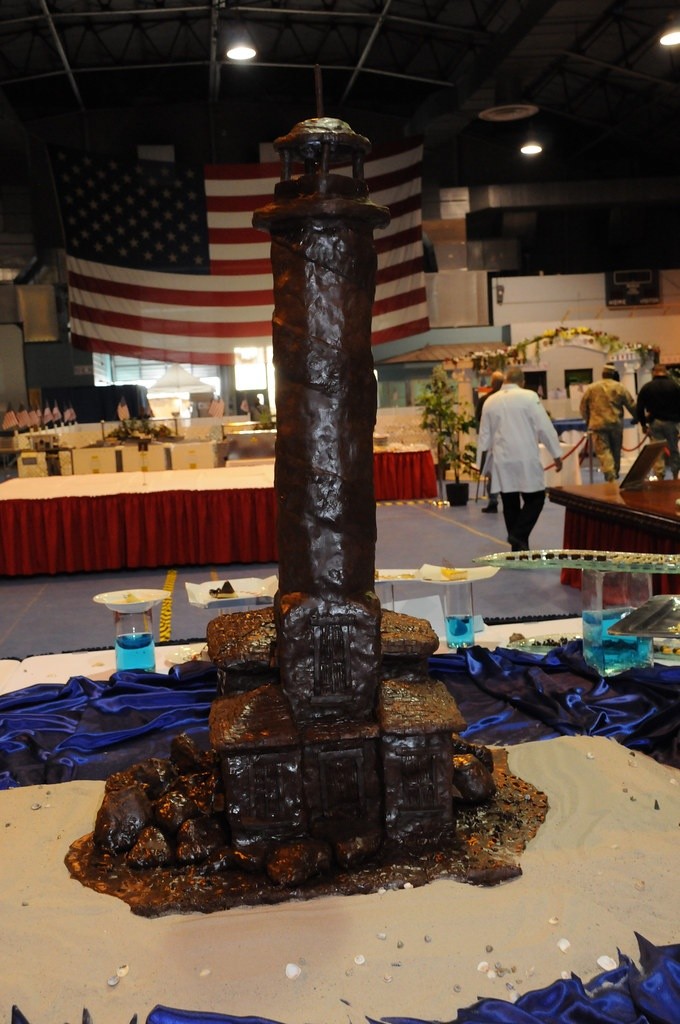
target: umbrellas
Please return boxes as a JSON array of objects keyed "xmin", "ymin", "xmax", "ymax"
[{"xmin": 146, "ymin": 365, "xmax": 213, "ymax": 394}]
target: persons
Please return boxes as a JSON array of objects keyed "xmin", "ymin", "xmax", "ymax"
[
  {"xmin": 580, "ymin": 362, "xmax": 637, "ymax": 483},
  {"xmin": 478, "ymin": 371, "xmax": 503, "ymax": 512},
  {"xmin": 637, "ymin": 365, "xmax": 680, "ymax": 481},
  {"xmin": 253, "ymin": 399, "xmax": 264, "ymax": 421},
  {"xmin": 477, "ymin": 366, "xmax": 563, "ymax": 558}
]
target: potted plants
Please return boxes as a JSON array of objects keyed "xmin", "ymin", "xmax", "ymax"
[{"xmin": 419, "ymin": 364, "xmax": 477, "ymax": 506}]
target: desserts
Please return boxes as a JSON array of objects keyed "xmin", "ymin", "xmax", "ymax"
[{"xmin": 63, "ymin": 116, "xmax": 549, "ymax": 916}]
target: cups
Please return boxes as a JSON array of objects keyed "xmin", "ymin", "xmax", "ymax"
[
  {"xmin": 116, "ymin": 613, "xmax": 156, "ymax": 674},
  {"xmin": 445, "ymin": 583, "xmax": 476, "ymax": 649},
  {"xmin": 582, "ymin": 567, "xmax": 654, "ymax": 677}
]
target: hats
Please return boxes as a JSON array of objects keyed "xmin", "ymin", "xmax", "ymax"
[{"xmin": 603, "ymin": 362, "xmax": 617, "ymax": 377}]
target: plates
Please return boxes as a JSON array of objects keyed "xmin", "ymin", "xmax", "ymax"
[
  {"xmin": 474, "ymin": 550, "xmax": 680, "ymax": 573},
  {"xmin": 185, "ymin": 575, "xmax": 278, "ymax": 609},
  {"xmin": 511, "ymin": 633, "xmax": 580, "ymax": 653},
  {"xmin": 415, "ymin": 564, "xmax": 501, "ymax": 585},
  {"xmin": 93, "ymin": 589, "xmax": 171, "ymax": 613},
  {"xmin": 375, "ymin": 569, "xmax": 424, "ymax": 582}
]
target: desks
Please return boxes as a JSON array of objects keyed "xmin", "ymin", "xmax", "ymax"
[
  {"xmin": 0, "ymin": 464, "xmax": 277, "ymax": 576},
  {"xmin": 546, "ymin": 479, "xmax": 680, "ymax": 605},
  {"xmin": 373, "ymin": 444, "xmax": 438, "ymax": 503},
  {"xmin": 539, "ymin": 442, "xmax": 583, "ymax": 490}
]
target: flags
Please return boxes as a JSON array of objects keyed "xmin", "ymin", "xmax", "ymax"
[
  {"xmin": 47, "ymin": 131, "xmax": 428, "ymax": 365},
  {"xmin": 3, "ymin": 399, "xmax": 75, "ymax": 430},
  {"xmin": 240, "ymin": 400, "xmax": 249, "ymax": 412},
  {"xmin": 117, "ymin": 397, "xmax": 130, "ymax": 421},
  {"xmin": 209, "ymin": 396, "xmax": 224, "ymax": 421}
]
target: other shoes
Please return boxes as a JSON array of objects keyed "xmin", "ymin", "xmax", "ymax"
[
  {"xmin": 615, "ymin": 475, "xmax": 620, "ymax": 479},
  {"xmin": 481, "ymin": 503, "xmax": 498, "ymax": 513}
]
